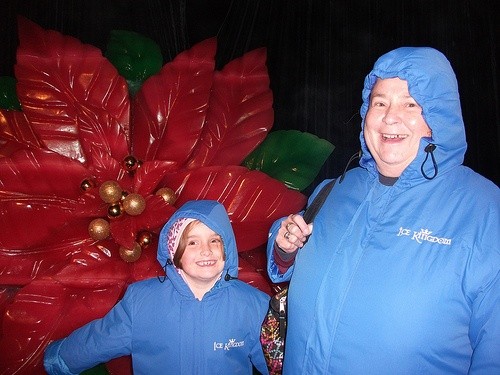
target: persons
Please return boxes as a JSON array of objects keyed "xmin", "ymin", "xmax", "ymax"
[
  {"xmin": 274, "ymin": 47, "xmax": 500, "ymax": 375},
  {"xmin": 44, "ymin": 200, "xmax": 271, "ymax": 375}
]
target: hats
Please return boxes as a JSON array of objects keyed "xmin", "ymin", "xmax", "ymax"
[{"xmin": 167, "ymin": 218, "xmax": 197, "ymax": 269}]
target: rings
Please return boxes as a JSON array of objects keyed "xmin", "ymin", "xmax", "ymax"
[
  {"xmin": 284, "ymin": 232, "xmax": 291, "ymax": 238},
  {"xmin": 292, "ymin": 214, "xmax": 299, "ymax": 221},
  {"xmin": 286, "ymin": 222, "xmax": 295, "ymax": 231}
]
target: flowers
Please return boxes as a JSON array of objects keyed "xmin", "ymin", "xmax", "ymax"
[{"xmin": 0, "ymin": 15, "xmax": 336, "ymax": 375}]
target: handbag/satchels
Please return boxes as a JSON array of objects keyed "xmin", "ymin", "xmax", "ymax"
[{"xmin": 258, "ymin": 178, "xmax": 339, "ymax": 375}]
